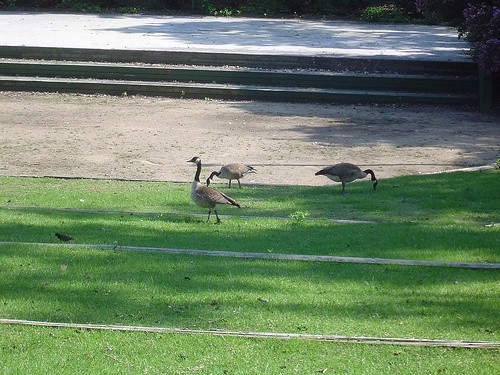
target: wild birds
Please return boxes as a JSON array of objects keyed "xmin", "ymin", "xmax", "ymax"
[
  {"xmin": 206, "ymin": 163, "xmax": 257, "ymax": 188},
  {"xmin": 54, "ymin": 233, "xmax": 75, "ymax": 243},
  {"xmin": 187, "ymin": 156, "xmax": 241, "ymax": 224},
  {"xmin": 315, "ymin": 162, "xmax": 378, "ymax": 192}
]
